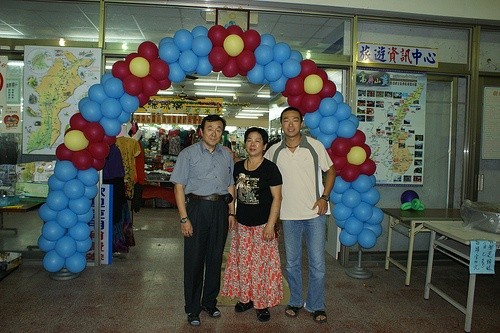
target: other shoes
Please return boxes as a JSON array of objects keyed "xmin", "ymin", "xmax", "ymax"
[
  {"xmin": 235, "ymin": 299, "xmax": 254, "ymax": 312},
  {"xmin": 256, "ymin": 307, "xmax": 271, "ymax": 322}
]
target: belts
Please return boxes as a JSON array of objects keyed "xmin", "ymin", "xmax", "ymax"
[{"xmin": 186, "ymin": 193, "xmax": 226, "ymax": 202}]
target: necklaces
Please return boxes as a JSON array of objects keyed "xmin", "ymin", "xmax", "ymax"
[
  {"xmin": 247, "ymin": 160, "xmax": 248, "ymax": 170},
  {"xmin": 285, "ymin": 144, "xmax": 298, "ymax": 148}
]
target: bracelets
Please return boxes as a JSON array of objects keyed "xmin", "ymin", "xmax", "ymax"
[
  {"xmin": 229, "ymin": 214, "xmax": 234, "ymax": 216},
  {"xmin": 322, "ymin": 195, "xmax": 329, "ymax": 200}
]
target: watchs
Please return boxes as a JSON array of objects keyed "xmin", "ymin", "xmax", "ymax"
[{"xmin": 180, "ymin": 217, "xmax": 189, "ymax": 223}]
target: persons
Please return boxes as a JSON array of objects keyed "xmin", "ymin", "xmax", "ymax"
[
  {"xmin": 222, "ymin": 107, "xmax": 335, "ymax": 322},
  {"xmin": 221, "ymin": 127, "xmax": 283, "ymax": 322},
  {"xmin": 169, "ymin": 115, "xmax": 235, "ymax": 326}
]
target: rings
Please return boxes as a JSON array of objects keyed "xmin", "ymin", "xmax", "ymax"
[{"xmin": 186, "ymin": 233, "xmax": 188, "ymax": 234}]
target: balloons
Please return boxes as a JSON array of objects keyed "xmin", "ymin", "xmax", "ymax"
[
  {"xmin": 159, "ymin": 26, "xmax": 212, "ymax": 82},
  {"xmin": 56, "ymin": 112, "xmax": 116, "ymax": 171},
  {"xmin": 304, "ymin": 91, "xmax": 358, "ymax": 148},
  {"xmin": 327, "ymin": 130, "xmax": 376, "ymax": 182},
  {"xmin": 282, "ymin": 60, "xmax": 336, "ymax": 116},
  {"xmin": 400, "ymin": 190, "xmax": 424, "ymax": 211},
  {"xmin": 38, "ymin": 160, "xmax": 98, "ymax": 274},
  {"xmin": 208, "ymin": 25, "xmax": 261, "ymax": 77},
  {"xmin": 330, "ymin": 174, "xmax": 384, "ymax": 248},
  {"xmin": 112, "ymin": 40, "xmax": 171, "ymax": 107},
  {"xmin": 247, "ymin": 34, "xmax": 303, "ymax": 92},
  {"xmin": 79, "ymin": 74, "xmax": 140, "ymax": 136}
]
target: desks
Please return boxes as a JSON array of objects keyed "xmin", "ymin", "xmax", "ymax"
[
  {"xmin": 380, "ymin": 208, "xmax": 462, "ymax": 285},
  {"xmin": 0, "ymin": 201, "xmax": 47, "ymax": 237},
  {"xmin": 423, "ymin": 221, "xmax": 500, "ymax": 332}
]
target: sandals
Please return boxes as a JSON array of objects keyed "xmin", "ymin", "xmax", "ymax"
[
  {"xmin": 284, "ymin": 304, "xmax": 303, "ymax": 317},
  {"xmin": 201, "ymin": 305, "xmax": 221, "ymax": 318},
  {"xmin": 313, "ymin": 309, "xmax": 327, "ymax": 323},
  {"xmin": 187, "ymin": 311, "xmax": 201, "ymax": 326}
]
